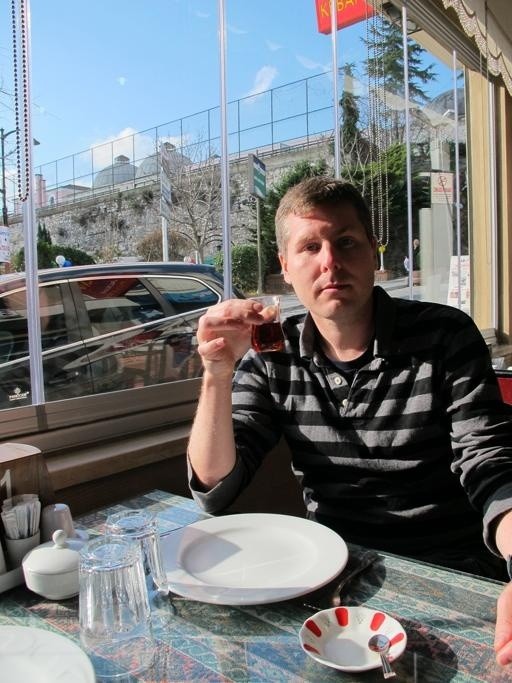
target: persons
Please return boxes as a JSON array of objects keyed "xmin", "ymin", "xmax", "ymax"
[{"xmin": 185, "ymin": 178, "xmax": 511, "ymax": 668}]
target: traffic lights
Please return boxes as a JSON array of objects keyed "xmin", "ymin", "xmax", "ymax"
[{"xmin": 160, "ymin": 142, "xmax": 170, "ymax": 161}]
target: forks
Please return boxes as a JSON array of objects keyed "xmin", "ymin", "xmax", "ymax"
[{"xmin": 301, "ymin": 551, "xmax": 381, "ymax": 614}]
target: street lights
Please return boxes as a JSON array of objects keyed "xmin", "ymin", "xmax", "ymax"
[{"xmin": 0, "ymin": 126, "xmax": 40, "ymax": 225}]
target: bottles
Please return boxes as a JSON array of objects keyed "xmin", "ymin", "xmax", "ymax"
[{"xmin": 43, "ymin": 502, "xmax": 78, "ymax": 540}]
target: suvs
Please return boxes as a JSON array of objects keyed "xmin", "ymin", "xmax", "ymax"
[{"xmin": 0, "ymin": 260, "xmax": 249, "ymax": 406}]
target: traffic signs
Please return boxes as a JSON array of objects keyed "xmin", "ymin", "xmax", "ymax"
[
  {"xmin": 159, "ymin": 198, "xmax": 173, "ymax": 224},
  {"xmin": 161, "ymin": 153, "xmax": 171, "ymax": 175},
  {"xmin": 159, "ymin": 169, "xmax": 173, "ymax": 205}
]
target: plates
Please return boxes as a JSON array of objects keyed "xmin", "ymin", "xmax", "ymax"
[
  {"xmin": 150, "ymin": 511, "xmax": 348, "ymax": 609},
  {"xmin": 296, "ymin": 600, "xmax": 407, "ymax": 673},
  {"xmin": 1, "ymin": 623, "xmax": 97, "ymax": 681}
]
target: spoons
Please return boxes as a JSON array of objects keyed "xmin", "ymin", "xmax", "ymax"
[{"xmin": 369, "ymin": 633, "xmax": 398, "ymax": 680}]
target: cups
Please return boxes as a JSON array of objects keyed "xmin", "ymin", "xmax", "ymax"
[
  {"xmin": 77, "ymin": 538, "xmax": 158, "ymax": 679},
  {"xmin": 6, "ymin": 529, "xmax": 44, "ymax": 567},
  {"xmin": 104, "ymin": 509, "xmax": 175, "ymax": 629},
  {"xmin": 22, "ymin": 529, "xmax": 88, "ymax": 602},
  {"xmin": 250, "ymin": 293, "xmax": 288, "ymax": 355}
]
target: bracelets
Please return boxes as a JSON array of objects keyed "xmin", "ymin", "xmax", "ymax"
[{"xmin": 506, "ymin": 554, "xmax": 510, "ymax": 576}]
